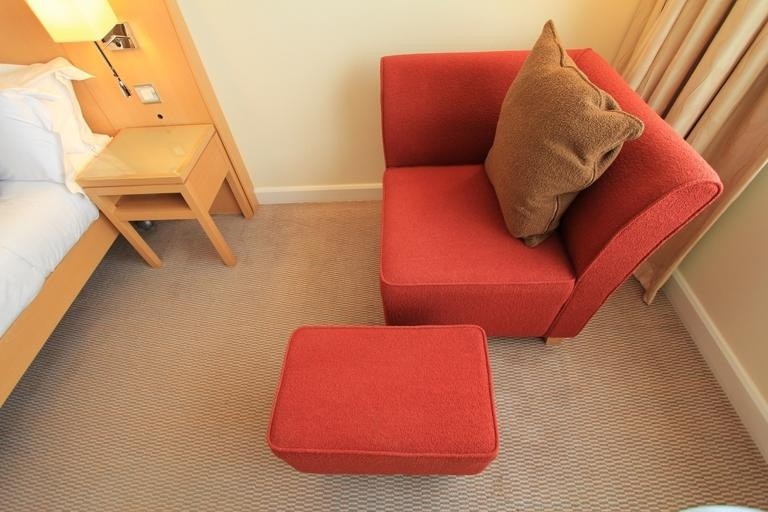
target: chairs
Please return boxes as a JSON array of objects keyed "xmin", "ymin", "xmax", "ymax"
[{"xmin": 379, "ymin": 48, "xmax": 725, "ymax": 346}]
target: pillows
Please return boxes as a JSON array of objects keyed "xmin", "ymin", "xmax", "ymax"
[
  {"xmin": 0, "ymin": 57, "xmax": 97, "ymax": 145},
  {"xmin": 0, "ymin": 89, "xmax": 95, "ymax": 198},
  {"xmin": 482, "ymin": 20, "xmax": 644, "ymax": 248}
]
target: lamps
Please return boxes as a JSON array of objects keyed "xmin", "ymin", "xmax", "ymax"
[{"xmin": 25, "ymin": 0, "xmax": 138, "ymax": 98}]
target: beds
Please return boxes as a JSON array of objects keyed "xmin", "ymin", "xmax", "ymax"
[{"xmin": 0, "ymin": 0, "xmax": 258, "ymax": 411}]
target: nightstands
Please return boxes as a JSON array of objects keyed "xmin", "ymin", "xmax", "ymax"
[{"xmin": 74, "ymin": 123, "xmax": 254, "ymax": 268}]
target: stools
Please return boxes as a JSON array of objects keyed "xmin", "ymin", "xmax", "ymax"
[{"xmin": 265, "ymin": 323, "xmax": 499, "ymax": 476}]
